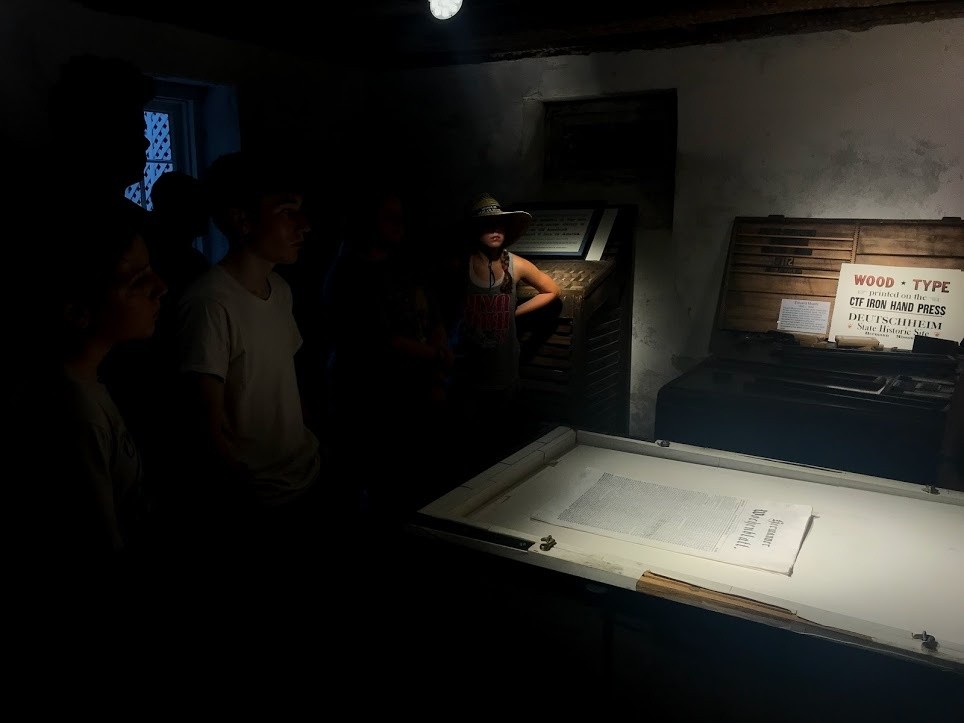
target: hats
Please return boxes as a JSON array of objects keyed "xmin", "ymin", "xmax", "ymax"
[{"xmin": 468, "ymin": 199, "xmax": 533, "ymax": 250}]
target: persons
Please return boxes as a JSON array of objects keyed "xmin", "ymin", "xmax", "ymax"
[{"xmin": 0, "ymin": 53, "xmax": 559, "ymax": 552}]
